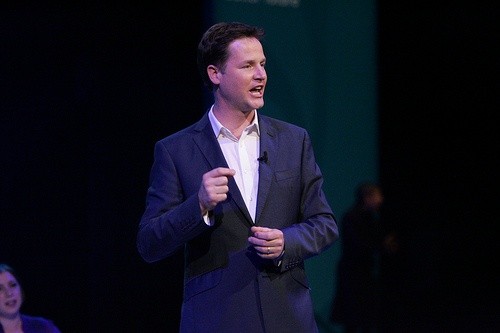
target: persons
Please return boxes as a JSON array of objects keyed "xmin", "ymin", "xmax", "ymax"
[
  {"xmin": 0, "ymin": 265, "xmax": 62, "ymax": 333},
  {"xmin": 135, "ymin": 21, "xmax": 339, "ymax": 333},
  {"xmin": 339, "ymin": 182, "xmax": 409, "ymax": 306}
]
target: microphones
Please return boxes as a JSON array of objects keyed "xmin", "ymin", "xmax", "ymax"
[{"xmin": 258, "ymin": 150, "xmax": 268, "ymax": 165}]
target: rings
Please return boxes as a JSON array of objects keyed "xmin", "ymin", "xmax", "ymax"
[{"xmin": 266, "ymin": 248, "xmax": 271, "ymax": 254}]
